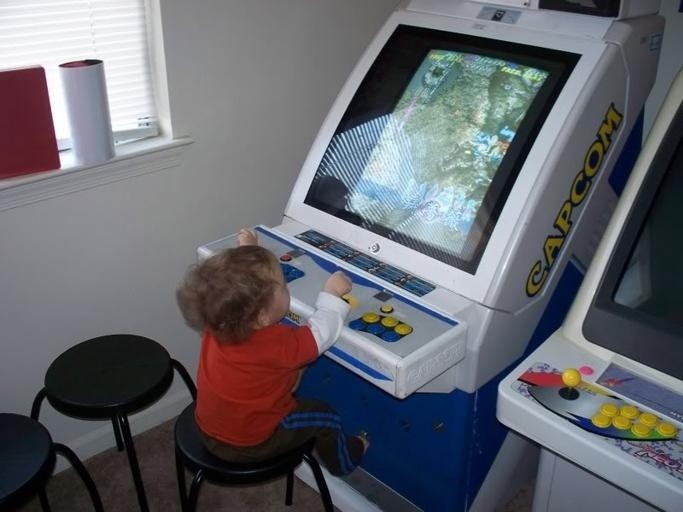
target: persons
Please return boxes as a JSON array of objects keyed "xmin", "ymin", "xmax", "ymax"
[{"xmin": 176, "ymin": 226, "xmax": 367, "ymax": 479}]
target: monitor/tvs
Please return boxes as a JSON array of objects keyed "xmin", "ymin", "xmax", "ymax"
[
  {"xmin": 604, "ymin": 123, "xmax": 683, "ymax": 329},
  {"xmin": 299, "ymin": 20, "xmax": 585, "ymax": 277}
]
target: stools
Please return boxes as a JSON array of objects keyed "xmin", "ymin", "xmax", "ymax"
[
  {"xmin": 28, "ymin": 334, "xmax": 197, "ymax": 510},
  {"xmin": 1, "ymin": 412, "xmax": 105, "ymax": 509},
  {"xmin": 175, "ymin": 392, "xmax": 334, "ymax": 508}
]
